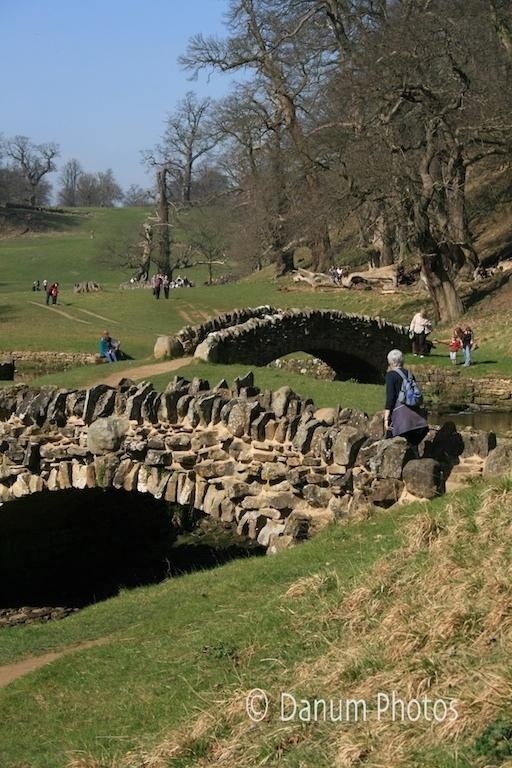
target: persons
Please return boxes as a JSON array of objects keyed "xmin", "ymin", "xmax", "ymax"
[
  {"xmin": 150, "ymin": 273, "xmax": 192, "ymax": 299},
  {"xmin": 449, "ymin": 327, "xmax": 474, "ymax": 367},
  {"xmin": 473, "ymin": 262, "xmax": 500, "ymax": 280},
  {"xmin": 383, "ymin": 349, "xmax": 428, "ymax": 459},
  {"xmin": 99, "ymin": 330, "xmax": 120, "ymax": 363},
  {"xmin": 409, "ymin": 307, "xmax": 433, "ymax": 357},
  {"xmin": 33, "ymin": 279, "xmax": 59, "ymax": 305},
  {"xmin": 329, "ymin": 266, "xmax": 348, "ymax": 279}
]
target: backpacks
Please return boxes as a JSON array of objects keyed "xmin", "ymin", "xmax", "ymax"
[{"xmin": 387, "ymin": 368, "xmax": 423, "ymax": 406}]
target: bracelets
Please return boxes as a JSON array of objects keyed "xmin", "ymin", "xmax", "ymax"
[{"xmin": 384, "ymin": 417, "xmax": 390, "ymax": 420}]
